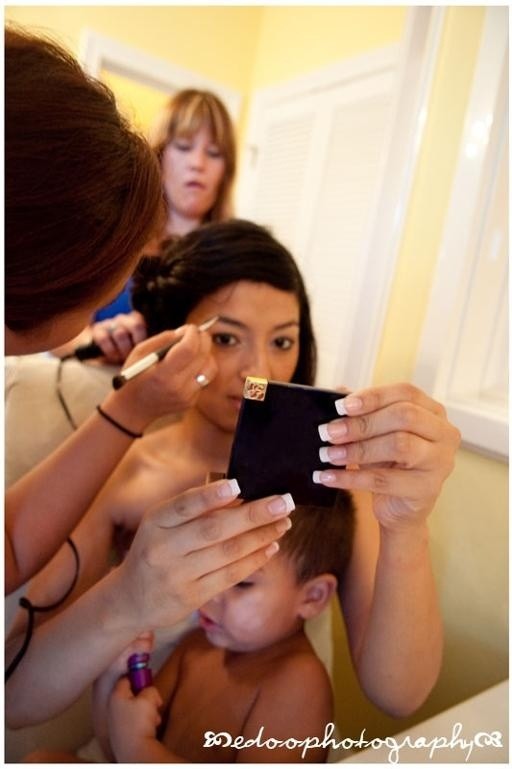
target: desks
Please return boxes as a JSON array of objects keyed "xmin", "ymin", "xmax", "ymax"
[{"xmin": 334, "ymin": 679, "xmax": 509, "ymax": 763}]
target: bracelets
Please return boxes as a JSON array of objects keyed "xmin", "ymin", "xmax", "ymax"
[{"xmin": 91, "ymin": 402, "xmax": 144, "ymax": 441}]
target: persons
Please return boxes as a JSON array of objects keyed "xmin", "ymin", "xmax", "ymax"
[
  {"xmin": 44, "ymin": 87, "xmax": 243, "ymax": 367},
  {"xmin": 6, "ymin": 19, "xmax": 222, "ymax": 600},
  {"xmin": 91, "ymin": 490, "xmax": 358, "ymax": 763},
  {"xmin": 4, "ymin": 214, "xmax": 460, "ymax": 763}
]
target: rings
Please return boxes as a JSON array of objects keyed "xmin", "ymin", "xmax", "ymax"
[
  {"xmin": 193, "ymin": 371, "xmax": 210, "ymax": 390},
  {"xmin": 107, "ymin": 320, "xmax": 121, "ymax": 335}
]
